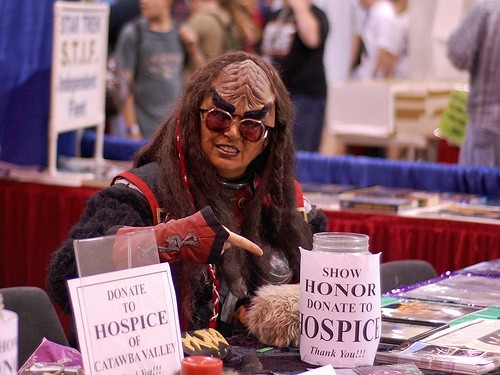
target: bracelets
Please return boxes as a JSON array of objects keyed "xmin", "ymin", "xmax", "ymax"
[{"xmin": 126, "ymin": 125, "xmax": 139, "ymax": 133}]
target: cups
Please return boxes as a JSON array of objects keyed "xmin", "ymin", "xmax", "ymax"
[{"xmin": 300, "ymin": 232, "xmax": 382, "ymax": 368}]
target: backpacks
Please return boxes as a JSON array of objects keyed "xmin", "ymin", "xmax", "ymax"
[{"xmin": 193, "ymin": 11, "xmax": 239, "ymax": 54}]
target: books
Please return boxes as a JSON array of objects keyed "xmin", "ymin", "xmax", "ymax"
[{"xmin": 339, "ymin": 186, "xmax": 500, "ymax": 220}]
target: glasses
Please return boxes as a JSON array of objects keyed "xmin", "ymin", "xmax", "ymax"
[{"xmin": 199, "ymin": 108, "xmax": 274, "ymax": 143}]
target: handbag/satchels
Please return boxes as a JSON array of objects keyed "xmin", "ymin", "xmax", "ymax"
[{"xmin": 106, "ymin": 21, "xmax": 140, "ymax": 117}]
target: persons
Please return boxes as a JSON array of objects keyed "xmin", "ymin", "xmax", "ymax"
[
  {"xmin": 46, "ymin": 50, "xmax": 327, "ymax": 351},
  {"xmin": 114, "ymin": 0, "xmax": 500, "ymax": 170}
]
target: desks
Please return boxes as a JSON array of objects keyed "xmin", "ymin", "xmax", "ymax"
[
  {"xmin": 0, "ymin": 164, "xmax": 500, "ymax": 375},
  {"xmin": 331, "ymin": 127, "xmax": 426, "ymax": 159}
]
